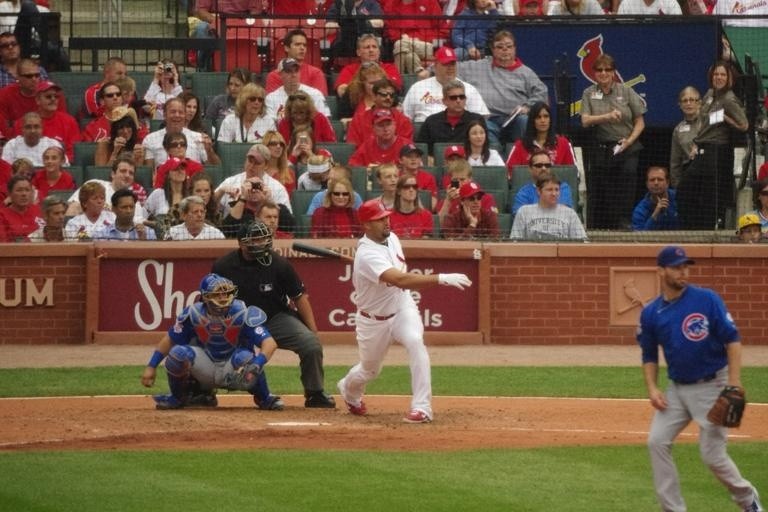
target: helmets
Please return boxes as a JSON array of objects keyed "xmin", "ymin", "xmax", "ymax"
[
  {"xmin": 359, "ymin": 200, "xmax": 390, "ymax": 222},
  {"xmin": 238, "ymin": 222, "xmax": 274, "ymax": 268},
  {"xmin": 201, "ymin": 273, "xmax": 239, "ymax": 316}
]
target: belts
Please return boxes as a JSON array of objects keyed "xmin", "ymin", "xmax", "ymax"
[
  {"xmin": 690, "ymin": 373, "xmax": 716, "ymax": 385},
  {"xmin": 360, "ymin": 311, "xmax": 396, "ymax": 320}
]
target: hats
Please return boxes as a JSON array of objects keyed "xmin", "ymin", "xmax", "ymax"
[
  {"xmin": 371, "ymin": 109, "xmax": 396, "ymax": 124},
  {"xmin": 277, "ymin": 59, "xmax": 299, "ymax": 73},
  {"xmin": 460, "ymin": 181, "xmax": 485, "ymax": 200},
  {"xmin": 657, "ymin": 247, "xmax": 695, "ymax": 267},
  {"xmin": 246, "ymin": 144, "xmax": 271, "ymax": 165},
  {"xmin": 399, "ymin": 144, "xmax": 424, "ymax": 157},
  {"xmin": 436, "ymin": 46, "xmax": 459, "ymax": 64},
  {"xmin": 104, "ymin": 106, "xmax": 139, "ymax": 129},
  {"xmin": 738, "ymin": 215, "xmax": 763, "ymax": 230},
  {"xmin": 445, "ymin": 145, "xmax": 463, "ymax": 159}
]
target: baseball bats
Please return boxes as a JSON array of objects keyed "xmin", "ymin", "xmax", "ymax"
[{"xmin": 293, "ymin": 243, "xmax": 354, "ymax": 264}]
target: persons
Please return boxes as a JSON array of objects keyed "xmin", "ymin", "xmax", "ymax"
[
  {"xmin": 1, "ymin": 1, "xmax": 768, "ymax": 245},
  {"xmin": 138, "ymin": 270, "xmax": 287, "ymax": 409},
  {"xmin": 334, "ymin": 196, "xmax": 473, "ymax": 425},
  {"xmin": 635, "ymin": 244, "xmax": 765, "ymax": 512},
  {"xmin": 208, "ymin": 216, "xmax": 339, "ymax": 409}
]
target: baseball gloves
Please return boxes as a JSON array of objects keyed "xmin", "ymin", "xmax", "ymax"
[
  {"xmin": 218, "ymin": 365, "xmax": 258, "ymax": 391},
  {"xmin": 707, "ymin": 385, "xmax": 747, "ymax": 427}
]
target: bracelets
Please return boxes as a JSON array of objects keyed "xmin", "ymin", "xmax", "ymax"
[{"xmin": 146, "ymin": 348, "xmax": 165, "ymax": 368}]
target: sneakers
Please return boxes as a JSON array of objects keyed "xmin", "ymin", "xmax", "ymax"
[
  {"xmin": 402, "ymin": 411, "xmax": 431, "ymax": 423},
  {"xmin": 304, "ymin": 390, "xmax": 337, "ymax": 408},
  {"xmin": 745, "ymin": 487, "xmax": 762, "ymax": 512},
  {"xmin": 186, "ymin": 392, "xmax": 218, "ymax": 408},
  {"xmin": 344, "ymin": 398, "xmax": 366, "ymax": 415},
  {"xmin": 255, "ymin": 397, "xmax": 284, "ymax": 410},
  {"xmin": 156, "ymin": 400, "xmax": 183, "ymax": 410}
]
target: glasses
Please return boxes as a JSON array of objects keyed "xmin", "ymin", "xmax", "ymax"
[
  {"xmin": 43, "ymin": 95, "xmax": 61, "ymax": 99},
  {"xmin": 289, "ymin": 95, "xmax": 305, "ymax": 101},
  {"xmin": 469, "ymin": 197, "xmax": 481, "ymax": 202},
  {"xmin": 0, "ymin": 41, "xmax": 18, "ymax": 49},
  {"xmin": 377, "ymin": 91, "xmax": 397, "ymax": 97},
  {"xmin": 168, "ymin": 142, "xmax": 186, "ymax": 148},
  {"xmin": 103, "ymin": 93, "xmax": 121, "ymax": 97},
  {"xmin": 595, "ymin": 68, "xmax": 613, "ymax": 71},
  {"xmin": 450, "ymin": 95, "xmax": 466, "ymax": 101},
  {"xmin": 268, "ymin": 141, "xmax": 284, "ymax": 147},
  {"xmin": 533, "ymin": 163, "xmax": 550, "ymax": 168},
  {"xmin": 247, "ymin": 96, "xmax": 262, "ymax": 102},
  {"xmin": 331, "ymin": 191, "xmax": 349, "ymax": 196},
  {"xmin": 400, "ymin": 184, "xmax": 418, "ymax": 190},
  {"xmin": 20, "ymin": 73, "xmax": 41, "ymax": 78}
]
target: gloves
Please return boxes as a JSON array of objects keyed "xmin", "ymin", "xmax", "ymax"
[{"xmin": 438, "ymin": 273, "xmax": 472, "ymax": 291}]
